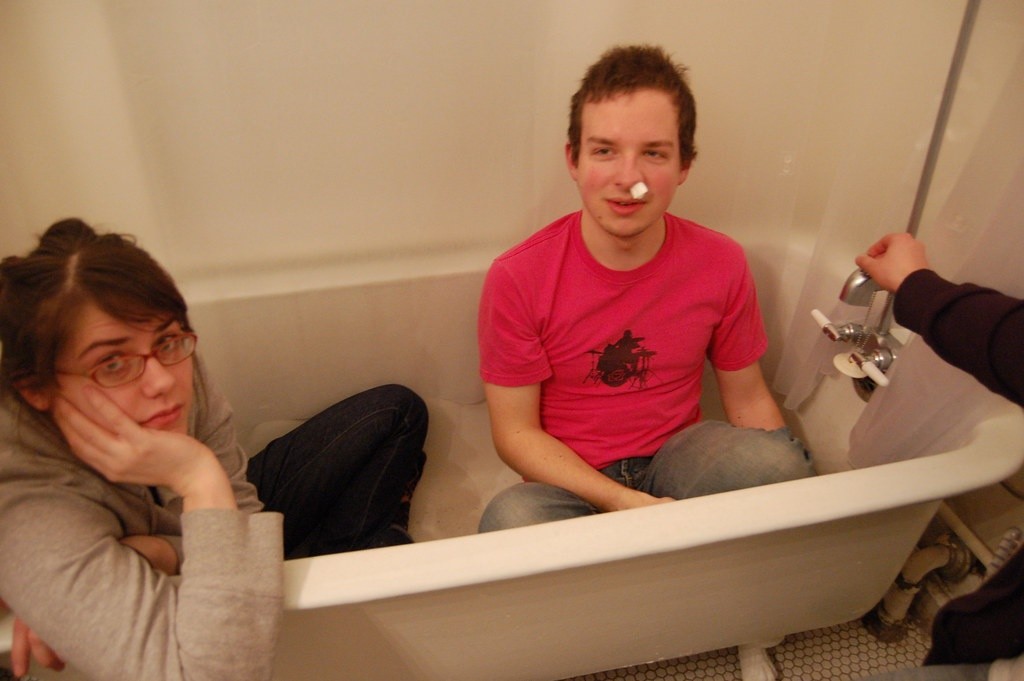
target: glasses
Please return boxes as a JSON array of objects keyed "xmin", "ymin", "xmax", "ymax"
[{"xmin": 54, "ymin": 333, "xmax": 197, "ymax": 388}]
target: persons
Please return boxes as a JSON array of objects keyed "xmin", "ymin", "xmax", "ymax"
[
  {"xmin": 0, "ymin": 218, "xmax": 428, "ymax": 681},
  {"xmin": 476, "ymin": 45, "xmax": 816, "ymax": 534},
  {"xmin": 857, "ymin": 233, "xmax": 1024, "ymax": 670}
]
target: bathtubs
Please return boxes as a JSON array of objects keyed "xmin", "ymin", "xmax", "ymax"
[{"xmin": 1, "ymin": 215, "xmax": 1023, "ymax": 681}]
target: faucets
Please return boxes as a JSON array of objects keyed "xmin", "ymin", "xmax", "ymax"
[{"xmin": 839, "ymin": 264, "xmax": 888, "ymax": 307}]
